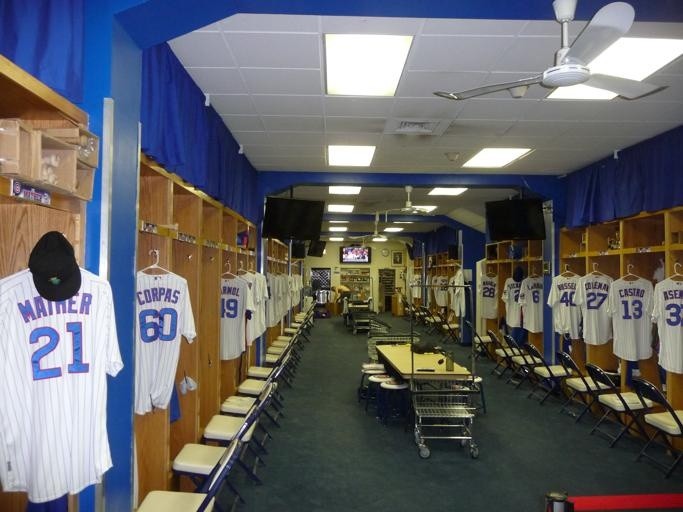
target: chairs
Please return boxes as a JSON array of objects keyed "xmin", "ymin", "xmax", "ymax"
[
  {"xmin": 399, "ymin": 294, "xmax": 460, "ymax": 345},
  {"xmin": 465, "ymin": 319, "xmax": 683, "ymax": 479},
  {"xmin": 134, "ymin": 298, "xmax": 318, "ymax": 511}
]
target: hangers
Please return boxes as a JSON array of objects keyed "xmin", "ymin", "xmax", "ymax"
[
  {"xmin": 411, "ymin": 261, "xmax": 683, "ymax": 284},
  {"xmin": 141, "ymin": 249, "xmax": 171, "ymax": 277},
  {"xmin": 221, "ymin": 260, "xmax": 301, "ymax": 278}
]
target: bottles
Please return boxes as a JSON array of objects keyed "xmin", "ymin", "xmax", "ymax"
[{"xmin": 445, "ymin": 344, "xmax": 455, "ymax": 371}]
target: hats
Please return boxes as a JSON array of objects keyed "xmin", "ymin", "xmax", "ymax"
[{"xmin": 27, "ymin": 231, "xmax": 82, "ymax": 302}]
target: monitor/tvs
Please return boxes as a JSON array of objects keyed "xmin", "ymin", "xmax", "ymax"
[
  {"xmin": 261, "ymin": 197, "xmax": 325, "ymax": 242},
  {"xmin": 308, "ymin": 241, "xmax": 326, "ymax": 257},
  {"xmin": 485, "ymin": 197, "xmax": 547, "ymax": 242},
  {"xmin": 340, "ymin": 246, "xmax": 371, "ymax": 263}
]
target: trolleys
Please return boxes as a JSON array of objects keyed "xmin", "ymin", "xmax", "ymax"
[
  {"xmin": 402, "ymin": 281, "xmax": 484, "ymax": 458},
  {"xmin": 347, "ymin": 300, "xmax": 390, "ymax": 337}
]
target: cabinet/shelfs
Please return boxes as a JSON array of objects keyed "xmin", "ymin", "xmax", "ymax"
[
  {"xmin": 426, "ymin": 252, "xmax": 459, "ymax": 338},
  {"xmin": 134, "ymin": 152, "xmax": 257, "ymax": 507},
  {"xmin": 0, "ymin": 53, "xmax": 90, "ymax": 512},
  {"xmin": 558, "ymin": 205, "xmax": 683, "ymax": 458},
  {"xmin": 340, "ymin": 266, "xmax": 371, "ymax": 303},
  {"xmin": 266, "ymin": 238, "xmax": 304, "ymax": 350},
  {"xmin": 412, "ymin": 256, "xmax": 423, "ymax": 312},
  {"xmin": 484, "ymin": 236, "xmax": 544, "ymax": 388}
]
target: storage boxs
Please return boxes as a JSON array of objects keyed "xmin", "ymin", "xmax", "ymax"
[{"xmin": 1, "ymin": 115, "xmax": 100, "ymax": 201}]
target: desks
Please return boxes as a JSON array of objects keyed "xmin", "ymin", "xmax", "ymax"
[
  {"xmin": 391, "ymin": 295, "xmax": 403, "ymax": 316},
  {"xmin": 377, "ymin": 342, "xmax": 471, "ymax": 432},
  {"xmin": 348, "ymin": 302, "xmax": 368, "ymax": 319}
]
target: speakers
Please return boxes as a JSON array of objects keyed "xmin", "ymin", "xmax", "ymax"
[{"xmin": 292, "ymin": 244, "xmax": 305, "ymax": 258}]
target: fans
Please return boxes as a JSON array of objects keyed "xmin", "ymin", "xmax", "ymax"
[
  {"xmin": 433, "ymin": 0, "xmax": 670, "ymax": 99},
  {"xmin": 384, "ymin": 185, "xmax": 432, "ymax": 216}
]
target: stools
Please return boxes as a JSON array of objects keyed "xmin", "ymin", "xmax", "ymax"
[
  {"xmin": 456, "ymin": 366, "xmax": 486, "ymax": 414},
  {"xmin": 359, "ymin": 361, "xmax": 410, "ymax": 426}
]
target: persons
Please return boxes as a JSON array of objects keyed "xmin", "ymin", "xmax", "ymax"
[
  {"xmin": 343, "ymin": 248, "xmax": 369, "ymax": 262},
  {"xmin": 329, "ymin": 285, "xmax": 351, "ymax": 316}
]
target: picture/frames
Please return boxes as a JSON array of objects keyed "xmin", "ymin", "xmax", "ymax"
[{"xmin": 391, "ymin": 249, "xmax": 404, "ymax": 267}]
